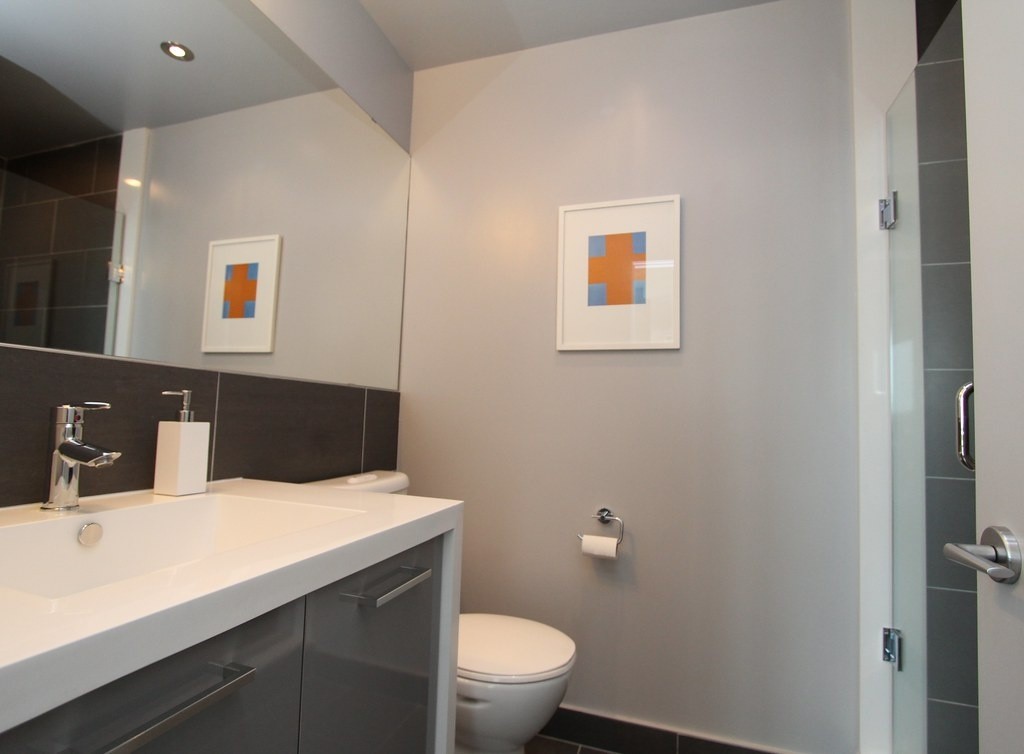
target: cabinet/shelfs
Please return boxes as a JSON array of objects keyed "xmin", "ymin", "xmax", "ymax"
[{"xmin": 0, "ymin": 534, "xmax": 445, "ymax": 754}]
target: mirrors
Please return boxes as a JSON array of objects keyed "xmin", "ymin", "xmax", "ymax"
[{"xmin": 0, "ymin": 0, "xmax": 411, "ymax": 393}]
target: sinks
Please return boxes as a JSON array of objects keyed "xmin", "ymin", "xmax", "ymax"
[{"xmin": 1, "ymin": 495, "xmax": 364, "ymax": 649}]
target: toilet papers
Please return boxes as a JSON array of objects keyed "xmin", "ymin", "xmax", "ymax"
[{"xmin": 582, "ymin": 535, "xmax": 619, "ymax": 560}]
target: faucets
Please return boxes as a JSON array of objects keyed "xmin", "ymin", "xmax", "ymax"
[{"xmin": 40, "ymin": 401, "xmax": 123, "ymax": 509}]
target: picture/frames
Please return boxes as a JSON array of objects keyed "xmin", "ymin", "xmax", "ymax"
[
  {"xmin": 556, "ymin": 195, "xmax": 680, "ymax": 351},
  {"xmin": 202, "ymin": 234, "xmax": 282, "ymax": 353}
]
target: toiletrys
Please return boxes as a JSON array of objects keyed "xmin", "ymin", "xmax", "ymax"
[{"xmin": 154, "ymin": 389, "xmax": 210, "ymax": 496}]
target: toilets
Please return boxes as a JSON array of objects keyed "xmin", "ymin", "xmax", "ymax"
[{"xmin": 305, "ymin": 469, "xmax": 577, "ymax": 753}]
